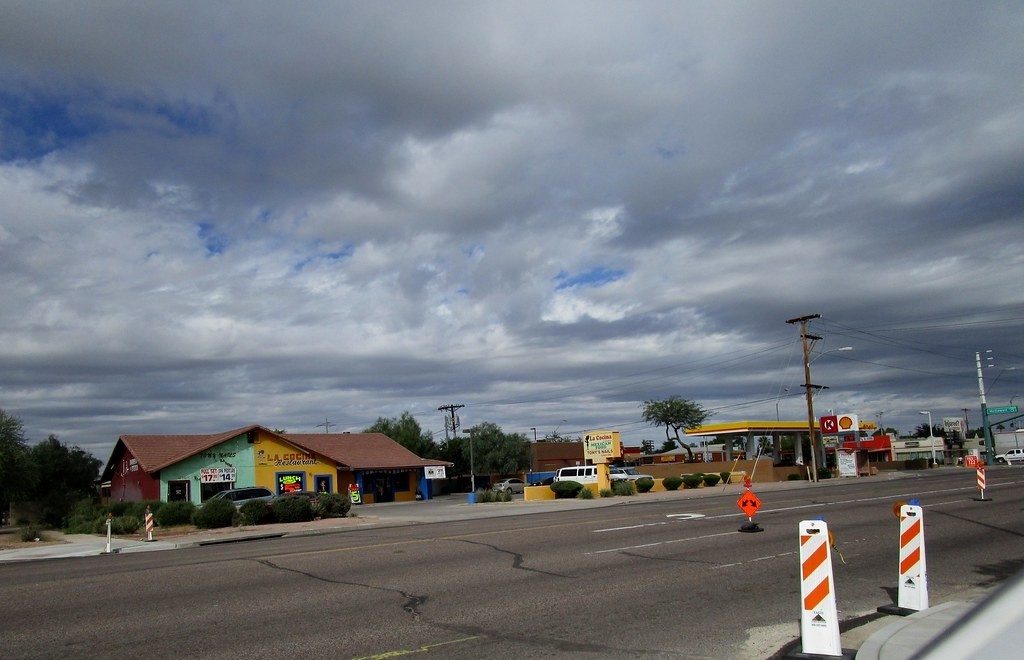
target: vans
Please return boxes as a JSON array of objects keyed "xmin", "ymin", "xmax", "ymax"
[
  {"xmin": 194, "ymin": 486, "xmax": 275, "ymax": 510},
  {"xmin": 555, "ymin": 465, "xmax": 625, "ymax": 485}
]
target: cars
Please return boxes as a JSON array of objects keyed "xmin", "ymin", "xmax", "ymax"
[
  {"xmin": 492, "ymin": 478, "xmax": 532, "ymax": 494},
  {"xmin": 553, "ymin": 469, "xmax": 560, "ymax": 482},
  {"xmin": 617, "ymin": 467, "xmax": 629, "ymax": 483},
  {"xmin": 266, "ymin": 491, "xmax": 343, "ymax": 508},
  {"xmin": 994, "ymin": 449, "xmax": 1024, "ymax": 463},
  {"xmin": 532, "ymin": 477, "xmax": 554, "ymax": 486},
  {"xmin": 914, "ymin": 457, "xmax": 940, "ymax": 468},
  {"xmin": 621, "ymin": 466, "xmax": 653, "ymax": 481}
]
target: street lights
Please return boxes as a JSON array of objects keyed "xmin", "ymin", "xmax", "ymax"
[
  {"xmin": 804, "ymin": 346, "xmax": 852, "ymax": 480},
  {"xmin": 1009, "ymin": 394, "xmax": 1021, "ymax": 449},
  {"xmin": 980, "ymin": 367, "xmax": 1017, "ymax": 465},
  {"xmin": 876, "ymin": 411, "xmax": 884, "ymax": 436},
  {"xmin": 919, "ymin": 412, "xmax": 939, "ymax": 469},
  {"xmin": 530, "ymin": 427, "xmax": 537, "ymax": 443},
  {"xmin": 462, "ymin": 428, "xmax": 482, "ymax": 493},
  {"xmin": 776, "ymin": 388, "xmax": 789, "ymax": 421},
  {"xmin": 961, "ymin": 408, "xmax": 970, "ymax": 438}
]
